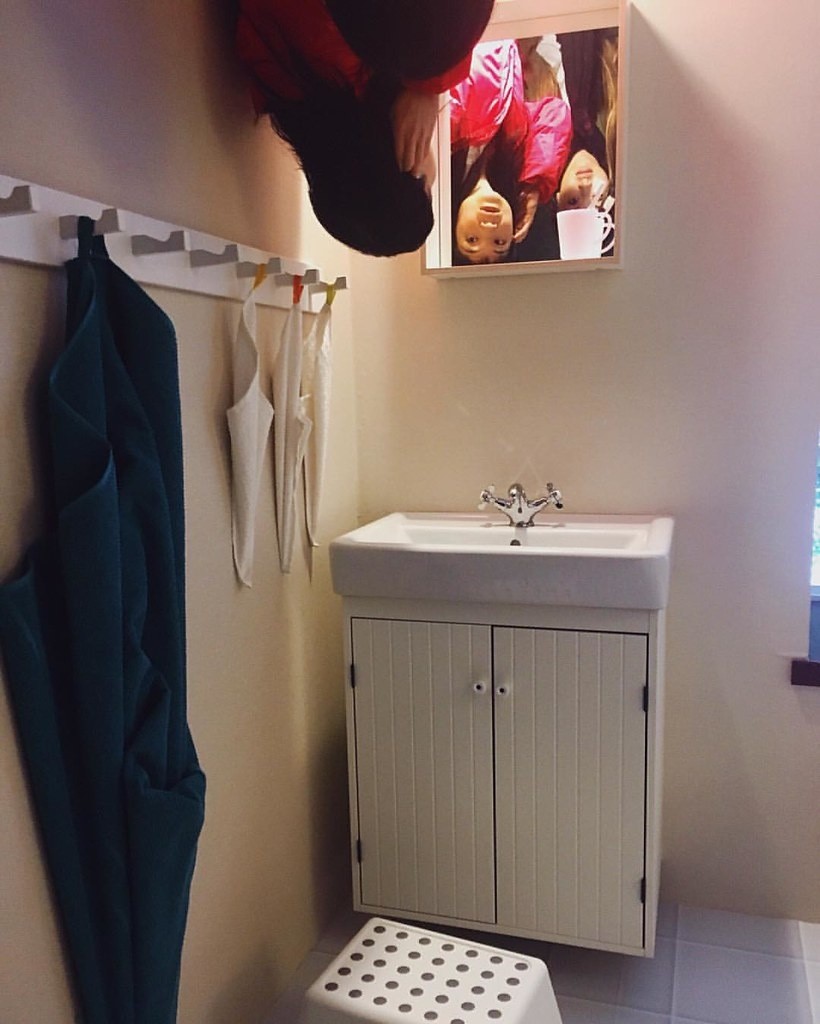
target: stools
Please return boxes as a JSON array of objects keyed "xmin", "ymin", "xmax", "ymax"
[{"xmin": 297, "ymin": 917, "xmax": 564, "ymax": 1024}]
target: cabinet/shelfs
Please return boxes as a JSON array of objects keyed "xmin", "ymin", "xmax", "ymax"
[{"xmin": 342, "ymin": 595, "xmax": 666, "ymax": 960}]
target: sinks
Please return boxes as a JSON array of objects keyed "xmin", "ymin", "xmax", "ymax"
[{"xmin": 328, "ymin": 513, "xmax": 677, "ymax": 610}]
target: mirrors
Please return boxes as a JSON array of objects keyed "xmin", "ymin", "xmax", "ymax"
[{"xmin": 420, "ymin": 0, "xmax": 633, "ymax": 279}]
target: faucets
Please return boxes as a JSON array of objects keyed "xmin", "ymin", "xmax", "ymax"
[{"xmin": 477, "ymin": 480, "xmax": 565, "ymax": 527}]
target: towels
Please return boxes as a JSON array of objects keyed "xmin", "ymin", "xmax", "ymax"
[
  {"xmin": 222, "ymin": 285, "xmax": 339, "ymax": 593},
  {"xmin": 0, "ymin": 210, "xmax": 209, "ymax": 1024}
]
